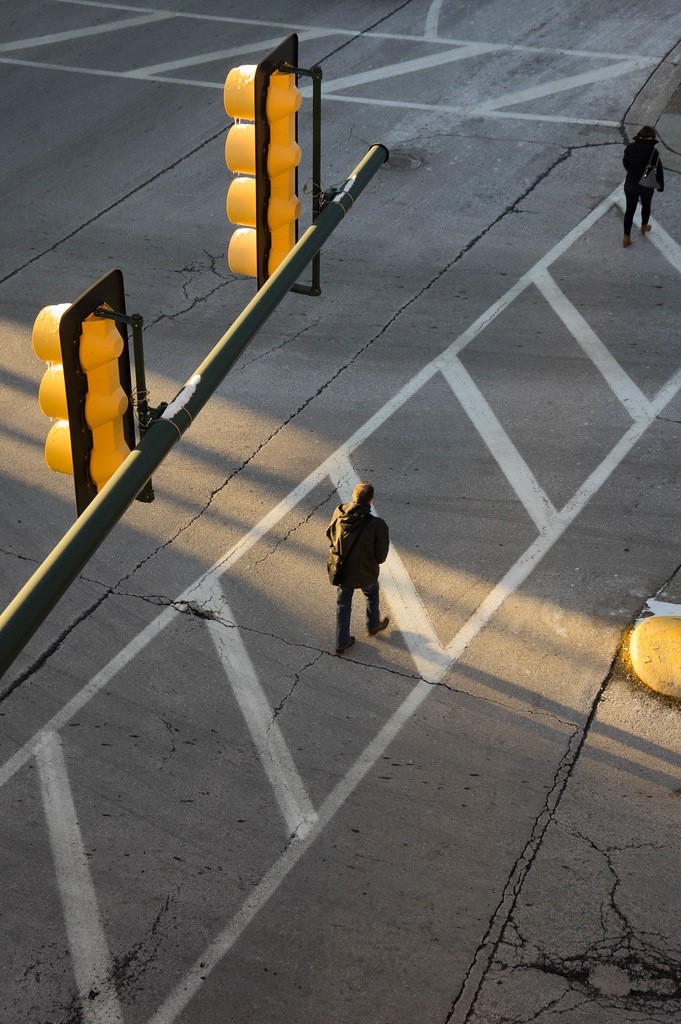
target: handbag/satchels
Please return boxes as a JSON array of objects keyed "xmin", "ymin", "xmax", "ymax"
[
  {"xmin": 639, "ymin": 148, "xmax": 661, "ymax": 189},
  {"xmin": 326, "ymin": 551, "xmax": 345, "ymax": 587}
]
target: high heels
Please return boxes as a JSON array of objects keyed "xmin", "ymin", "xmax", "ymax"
[{"xmin": 641, "ymin": 224, "xmax": 651, "ymax": 236}]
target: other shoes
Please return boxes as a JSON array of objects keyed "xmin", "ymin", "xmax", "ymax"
[
  {"xmin": 335, "ymin": 636, "xmax": 356, "ymax": 655},
  {"xmin": 368, "ymin": 616, "xmax": 389, "ymax": 636},
  {"xmin": 623, "ymin": 234, "xmax": 632, "ymax": 248}
]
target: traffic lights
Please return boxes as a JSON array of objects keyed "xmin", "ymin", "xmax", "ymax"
[
  {"xmin": 223, "ymin": 31, "xmax": 301, "ymax": 292},
  {"xmin": 31, "ymin": 268, "xmax": 138, "ymax": 519}
]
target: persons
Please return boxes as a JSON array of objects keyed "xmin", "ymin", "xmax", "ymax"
[
  {"xmin": 622, "ymin": 125, "xmax": 664, "ymax": 248},
  {"xmin": 324, "ymin": 482, "xmax": 390, "ymax": 654}
]
